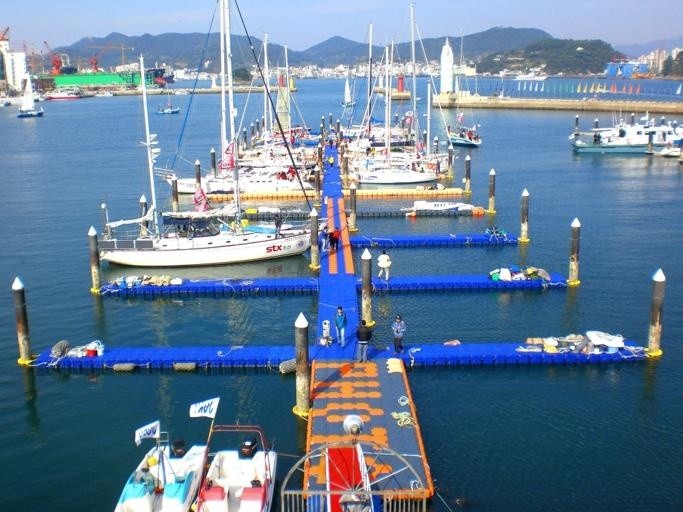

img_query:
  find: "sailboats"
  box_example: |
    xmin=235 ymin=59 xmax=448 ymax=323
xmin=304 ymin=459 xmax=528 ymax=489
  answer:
xmin=16 ymin=72 xmax=44 ymax=117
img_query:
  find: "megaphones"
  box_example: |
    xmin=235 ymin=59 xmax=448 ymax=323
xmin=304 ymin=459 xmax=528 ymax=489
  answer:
xmin=343 ymin=415 xmax=363 ymax=435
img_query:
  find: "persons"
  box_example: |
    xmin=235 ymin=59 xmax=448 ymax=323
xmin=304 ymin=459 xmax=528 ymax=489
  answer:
xmin=390 ymin=315 xmax=407 ymax=355
xmin=355 ymin=320 xmax=373 ymax=365
xmin=333 ymin=305 xmax=348 ymax=349
xmin=274 ymin=214 xmax=285 ymax=239
xmin=378 ymin=249 xmax=393 ymax=282
xmin=320 ymin=225 xmax=342 ymax=252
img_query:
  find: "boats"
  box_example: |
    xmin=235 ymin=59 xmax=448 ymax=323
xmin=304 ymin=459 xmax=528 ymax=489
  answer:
xmin=568 ymin=108 xmax=683 ymax=153
xmin=46 ymin=86 xmax=114 ymax=99
xmin=112 ymin=393 xmax=279 ymax=512
